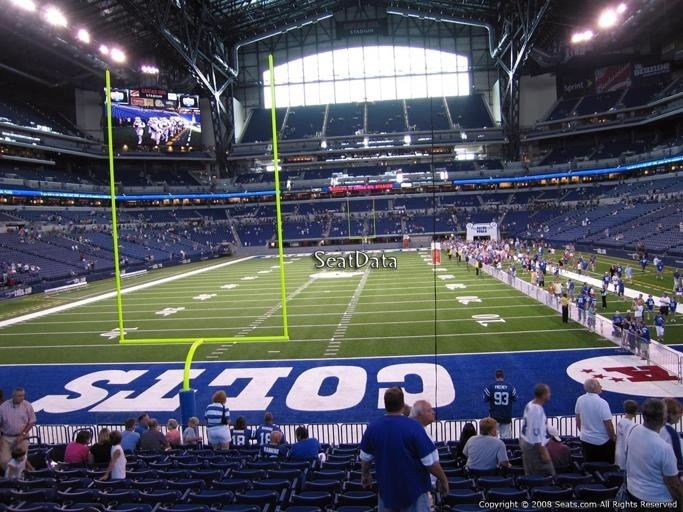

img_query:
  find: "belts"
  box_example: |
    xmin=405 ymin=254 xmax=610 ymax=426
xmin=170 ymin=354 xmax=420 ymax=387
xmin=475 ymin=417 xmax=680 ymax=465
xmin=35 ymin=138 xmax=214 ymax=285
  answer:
xmin=4 ymin=435 xmax=18 ymax=437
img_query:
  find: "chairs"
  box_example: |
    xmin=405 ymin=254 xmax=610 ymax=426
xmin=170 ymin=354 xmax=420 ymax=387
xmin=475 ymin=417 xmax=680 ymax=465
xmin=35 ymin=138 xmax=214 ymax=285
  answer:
xmin=116 ymin=152 xmax=236 ymax=267
xmin=0 ymin=84 xmax=116 ymax=295
xmin=236 ymin=93 xmax=500 ymax=241
xmin=0 ymin=422 xmax=682 ymax=512
xmin=498 ymin=60 xmax=683 ymax=268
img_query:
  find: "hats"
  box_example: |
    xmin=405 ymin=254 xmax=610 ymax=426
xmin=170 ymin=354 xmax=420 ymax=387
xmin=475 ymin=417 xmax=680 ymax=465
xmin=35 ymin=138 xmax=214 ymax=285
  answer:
xmin=547 ymin=426 xmax=562 ymax=442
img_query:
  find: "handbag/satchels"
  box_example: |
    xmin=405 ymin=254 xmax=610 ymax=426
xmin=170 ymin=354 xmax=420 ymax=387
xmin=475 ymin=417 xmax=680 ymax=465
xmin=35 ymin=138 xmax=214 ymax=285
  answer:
xmin=222 ymin=405 xmax=228 ymax=426
xmin=613 ymin=424 xmax=639 ymax=512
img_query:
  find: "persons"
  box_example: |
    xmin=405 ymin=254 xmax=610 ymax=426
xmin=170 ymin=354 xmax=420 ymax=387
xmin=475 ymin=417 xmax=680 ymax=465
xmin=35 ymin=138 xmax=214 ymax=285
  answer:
xmin=132 ymin=116 xmax=183 ymax=147
